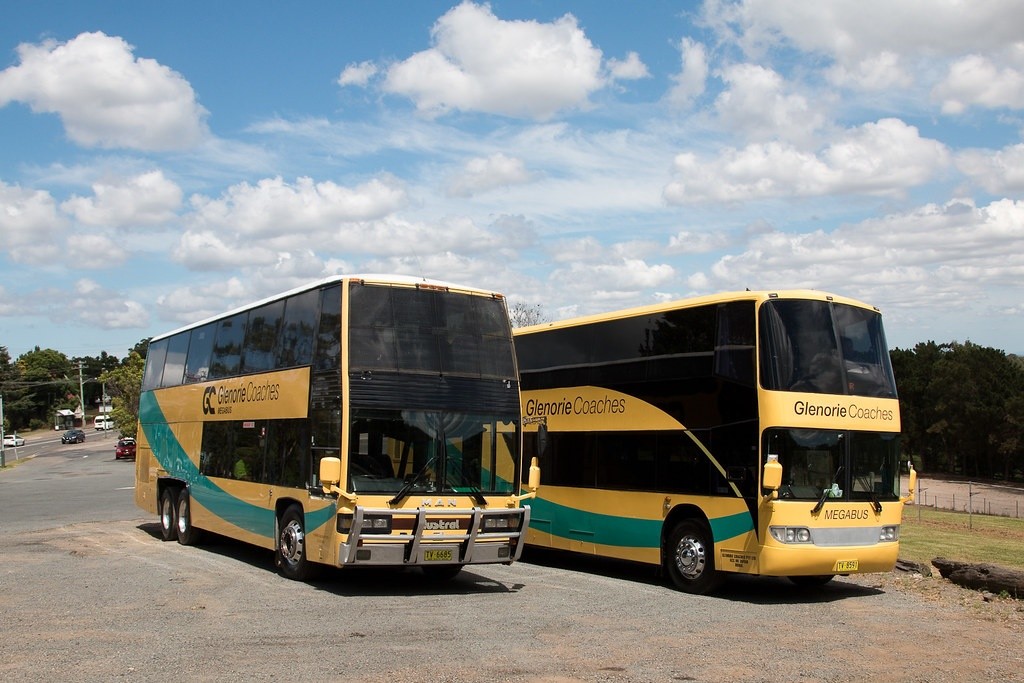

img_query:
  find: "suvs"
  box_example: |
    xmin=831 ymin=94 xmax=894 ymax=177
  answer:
xmin=61 ymin=429 xmax=86 ymax=444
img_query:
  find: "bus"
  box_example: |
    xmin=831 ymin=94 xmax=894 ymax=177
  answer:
xmin=132 ymin=272 xmax=542 ymax=584
xmin=511 ymin=289 xmax=917 ymax=595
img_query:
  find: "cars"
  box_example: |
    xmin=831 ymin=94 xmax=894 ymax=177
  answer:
xmin=3 ymin=434 xmax=25 ymax=447
xmin=114 ymin=437 xmax=137 ymax=460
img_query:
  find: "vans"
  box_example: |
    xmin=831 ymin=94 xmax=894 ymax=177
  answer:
xmin=94 ymin=415 xmax=115 ymax=431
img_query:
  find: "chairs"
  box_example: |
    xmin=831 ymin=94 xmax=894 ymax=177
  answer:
xmin=352 ymin=453 xmax=395 ymax=479
xmin=204 ymin=445 xmax=279 ymax=485
